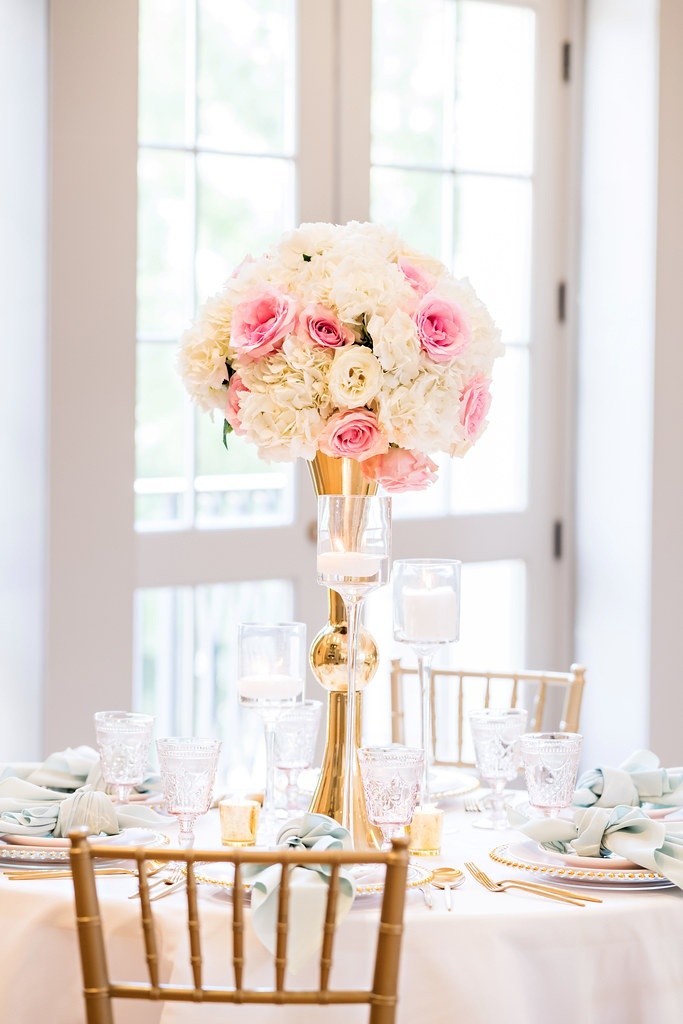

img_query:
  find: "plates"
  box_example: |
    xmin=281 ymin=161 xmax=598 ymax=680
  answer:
xmin=490 ymin=803 xmax=681 ymax=891
xmin=294 ymin=767 xmax=478 ymax=803
xmin=181 ymin=864 xmax=434 ymax=908
xmin=0 ymin=784 xmax=170 ymax=863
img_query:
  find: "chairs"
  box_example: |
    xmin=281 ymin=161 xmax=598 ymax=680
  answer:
xmin=390 ymin=659 xmax=588 ymax=774
xmin=64 ymin=826 xmax=414 ymax=1024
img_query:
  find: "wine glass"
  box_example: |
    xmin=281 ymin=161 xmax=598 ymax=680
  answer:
xmin=357 ymin=750 xmax=424 ymax=849
xmin=469 ymin=710 xmax=528 ymax=803
xmin=521 ymin=732 xmax=581 ymax=817
xmin=238 ymin=622 xmax=323 ymax=838
xmin=94 ymin=710 xmax=154 ymax=805
xmin=157 ymin=736 xmax=221 ymax=850
xmin=313 ymin=494 xmax=393 ymax=850
xmin=390 ymin=559 xmax=460 ymax=808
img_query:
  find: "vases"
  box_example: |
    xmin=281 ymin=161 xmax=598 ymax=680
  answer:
xmin=307 ymin=450 xmax=384 ymax=852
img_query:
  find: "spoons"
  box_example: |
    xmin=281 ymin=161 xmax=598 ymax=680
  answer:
xmin=431 ymin=867 xmax=465 ymax=910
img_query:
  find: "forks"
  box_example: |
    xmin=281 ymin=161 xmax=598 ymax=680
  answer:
xmin=463 ymin=793 xmax=489 ymax=812
xmin=129 ymin=866 xmax=184 ymax=899
xmin=464 ymin=862 xmax=603 ymax=907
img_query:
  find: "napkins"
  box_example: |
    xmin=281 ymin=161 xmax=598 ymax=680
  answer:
xmin=0 ymin=745 xmax=179 ymax=838
xmin=569 ymin=749 xmax=683 ymax=806
xmin=241 ymin=812 xmax=356 ymax=973
xmin=518 ymin=804 xmax=683 ymax=890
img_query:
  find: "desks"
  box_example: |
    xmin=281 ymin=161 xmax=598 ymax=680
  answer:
xmin=0 ymin=767 xmax=683 ymax=1024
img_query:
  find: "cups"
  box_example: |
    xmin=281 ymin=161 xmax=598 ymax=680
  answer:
xmin=221 ymin=801 xmax=256 ymax=845
xmin=410 ymin=808 xmax=443 ymax=854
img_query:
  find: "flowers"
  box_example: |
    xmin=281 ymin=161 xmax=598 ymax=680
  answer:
xmin=172 ymin=215 xmax=502 ymax=491
